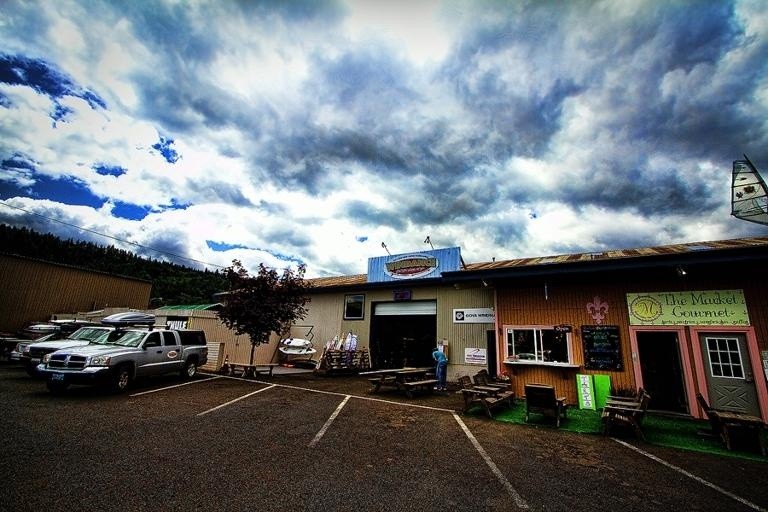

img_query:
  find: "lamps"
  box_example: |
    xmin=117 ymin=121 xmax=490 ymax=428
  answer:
xmin=423 ymin=234 xmax=436 ymax=251
xmin=380 ymin=240 xmax=392 ymax=256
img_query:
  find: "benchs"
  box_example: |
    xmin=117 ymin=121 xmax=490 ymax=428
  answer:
xmin=367 ymin=376 xmax=395 ymax=393
xmin=252 ymin=364 xmax=278 ymax=378
xmin=402 ymin=377 xmax=442 ymax=394
xmin=223 ymin=361 xmax=253 ymax=378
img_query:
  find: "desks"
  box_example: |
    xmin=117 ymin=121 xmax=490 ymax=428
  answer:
xmin=358 ymin=367 xmax=439 ymax=397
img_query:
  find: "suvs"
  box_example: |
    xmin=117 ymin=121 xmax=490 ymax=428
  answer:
xmin=0 ymin=311 xmax=208 ymax=393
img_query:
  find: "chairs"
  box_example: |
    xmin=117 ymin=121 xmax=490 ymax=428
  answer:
xmin=521 ymin=384 xmax=568 ymax=428
xmin=601 ymin=385 xmax=766 ymax=458
xmin=457 ymin=369 xmax=519 ymax=421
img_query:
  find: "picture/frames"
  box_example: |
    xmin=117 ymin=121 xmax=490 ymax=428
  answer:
xmin=343 ymin=293 xmax=366 ymax=320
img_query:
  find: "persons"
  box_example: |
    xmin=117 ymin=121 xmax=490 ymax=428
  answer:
xmin=431 ymin=344 xmax=450 ymax=392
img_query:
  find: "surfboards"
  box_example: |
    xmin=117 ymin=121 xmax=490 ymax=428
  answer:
xmin=278 ymin=329 xmax=358 ymax=372
